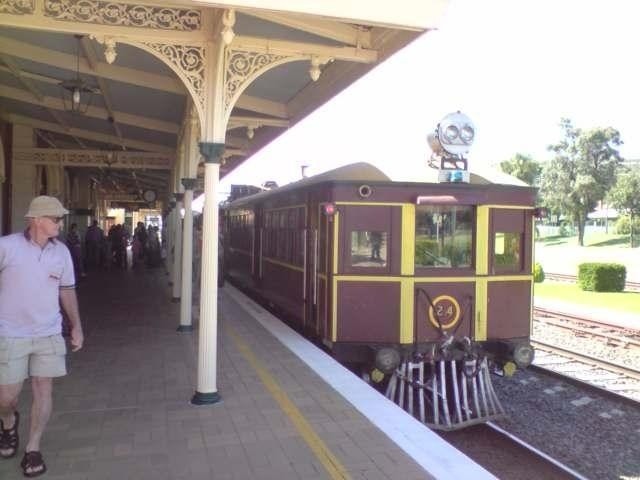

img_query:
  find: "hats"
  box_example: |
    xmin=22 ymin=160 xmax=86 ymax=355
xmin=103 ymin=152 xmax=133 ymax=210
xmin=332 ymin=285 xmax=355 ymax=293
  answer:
xmin=23 ymin=195 xmax=71 ymax=218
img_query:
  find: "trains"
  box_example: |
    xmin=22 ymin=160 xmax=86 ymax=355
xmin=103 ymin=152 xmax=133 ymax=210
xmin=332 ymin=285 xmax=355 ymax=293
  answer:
xmin=181 ymin=110 xmax=548 ymax=433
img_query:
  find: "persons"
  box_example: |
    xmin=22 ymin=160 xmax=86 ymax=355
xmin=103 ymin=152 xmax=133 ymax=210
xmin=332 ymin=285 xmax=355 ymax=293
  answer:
xmin=369 ymin=232 xmax=384 ymax=261
xmin=66 ymin=223 xmax=82 ymax=271
xmin=85 ymin=220 xmax=160 ymax=270
xmin=0 ymin=194 xmax=85 ymax=477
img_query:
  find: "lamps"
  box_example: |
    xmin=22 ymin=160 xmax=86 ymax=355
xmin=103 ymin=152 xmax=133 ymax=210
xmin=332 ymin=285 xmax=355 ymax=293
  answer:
xmin=100 ymin=114 xmax=118 ymax=173
xmin=59 ymin=35 xmax=102 ymax=124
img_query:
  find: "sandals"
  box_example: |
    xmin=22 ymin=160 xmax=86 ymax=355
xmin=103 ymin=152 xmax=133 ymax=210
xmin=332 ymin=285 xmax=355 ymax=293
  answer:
xmin=0 ymin=411 xmax=20 ymax=459
xmin=21 ymin=450 xmax=47 ymax=478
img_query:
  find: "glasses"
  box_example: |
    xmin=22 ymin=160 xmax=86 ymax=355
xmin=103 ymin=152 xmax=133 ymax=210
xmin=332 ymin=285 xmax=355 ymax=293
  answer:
xmin=40 ymin=216 xmax=63 ymax=224
